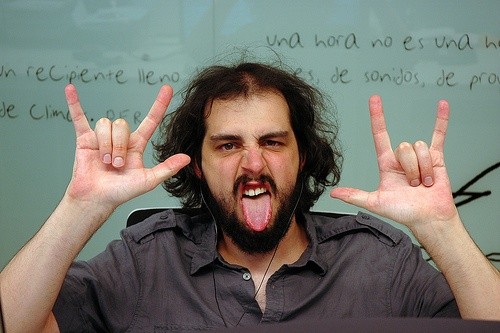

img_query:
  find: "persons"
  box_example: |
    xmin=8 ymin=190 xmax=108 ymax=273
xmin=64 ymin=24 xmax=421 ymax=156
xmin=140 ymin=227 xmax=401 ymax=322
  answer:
xmin=0 ymin=63 xmax=500 ymax=333
